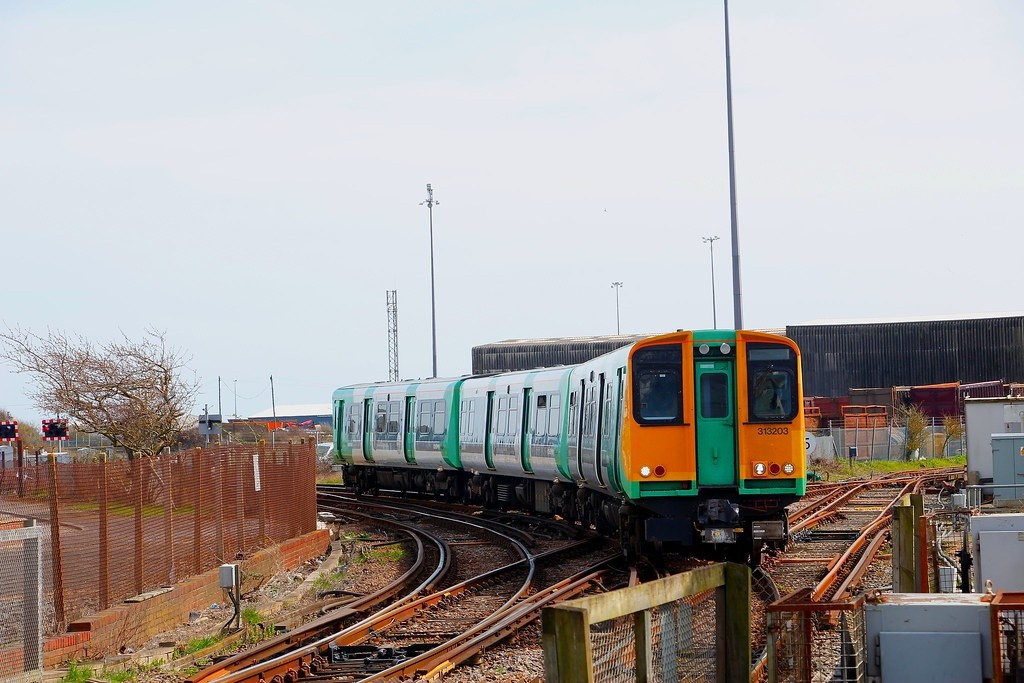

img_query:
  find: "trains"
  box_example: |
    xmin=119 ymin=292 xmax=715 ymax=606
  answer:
xmin=331 ymin=327 xmax=807 ymax=566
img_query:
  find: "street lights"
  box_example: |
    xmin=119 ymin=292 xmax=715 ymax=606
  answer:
xmin=419 ymin=183 xmax=440 ymax=377
xmin=702 ymin=235 xmax=721 ymax=330
xmin=610 ymin=282 xmax=624 ymax=335
